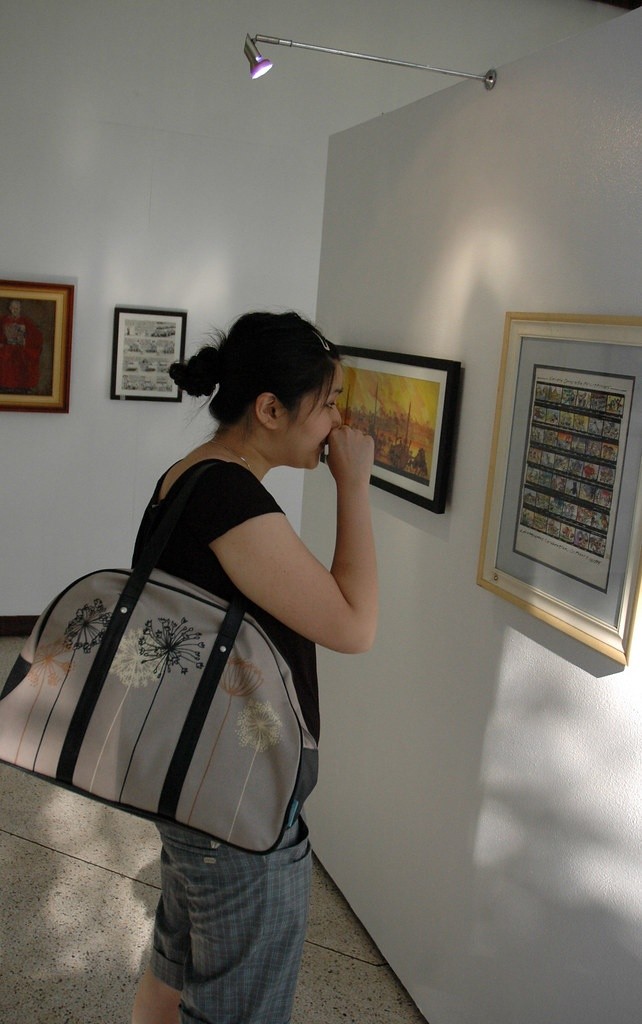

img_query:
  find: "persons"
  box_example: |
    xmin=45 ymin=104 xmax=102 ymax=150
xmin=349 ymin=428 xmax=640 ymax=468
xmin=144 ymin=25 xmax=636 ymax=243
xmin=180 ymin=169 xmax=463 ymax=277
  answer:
xmin=127 ymin=311 xmax=378 ymax=1024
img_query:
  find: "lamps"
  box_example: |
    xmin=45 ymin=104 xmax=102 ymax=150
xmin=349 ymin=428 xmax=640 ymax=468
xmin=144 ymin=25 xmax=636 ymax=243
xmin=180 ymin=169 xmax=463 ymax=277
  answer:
xmin=245 ymin=30 xmax=496 ymax=92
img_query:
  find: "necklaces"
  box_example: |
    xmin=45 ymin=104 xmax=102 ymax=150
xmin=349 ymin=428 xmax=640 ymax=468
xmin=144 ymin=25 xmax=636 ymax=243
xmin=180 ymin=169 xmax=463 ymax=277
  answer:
xmin=210 ymin=439 xmax=252 ymax=471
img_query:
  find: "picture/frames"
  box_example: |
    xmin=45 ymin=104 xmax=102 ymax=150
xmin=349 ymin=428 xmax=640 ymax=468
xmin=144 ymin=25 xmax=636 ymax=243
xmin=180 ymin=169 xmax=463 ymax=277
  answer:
xmin=0 ymin=277 xmax=75 ymax=414
xmin=475 ymin=312 xmax=642 ymax=668
xmin=318 ymin=344 xmax=465 ymax=515
xmin=109 ymin=305 xmax=188 ymax=404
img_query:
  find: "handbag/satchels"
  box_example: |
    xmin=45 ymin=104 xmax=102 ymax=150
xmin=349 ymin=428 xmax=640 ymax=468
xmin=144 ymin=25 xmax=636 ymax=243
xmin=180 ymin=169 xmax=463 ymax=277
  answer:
xmin=0 ymin=463 xmax=319 ymax=855
